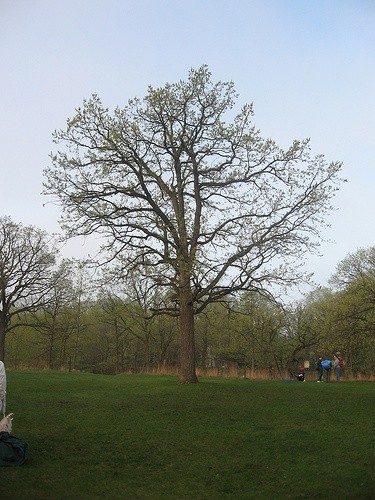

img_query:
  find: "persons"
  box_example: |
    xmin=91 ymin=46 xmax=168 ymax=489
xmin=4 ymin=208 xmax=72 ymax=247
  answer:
xmin=294 ymin=350 xmax=347 ymax=384
xmin=0 ymin=358 xmax=8 ymax=434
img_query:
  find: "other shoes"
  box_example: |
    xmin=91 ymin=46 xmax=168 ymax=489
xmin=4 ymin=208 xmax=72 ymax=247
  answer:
xmin=318 ymin=380 xmax=323 ymax=383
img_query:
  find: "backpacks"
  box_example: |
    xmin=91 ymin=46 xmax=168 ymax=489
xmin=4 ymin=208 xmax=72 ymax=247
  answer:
xmin=338 ymin=358 xmax=343 ymax=366
